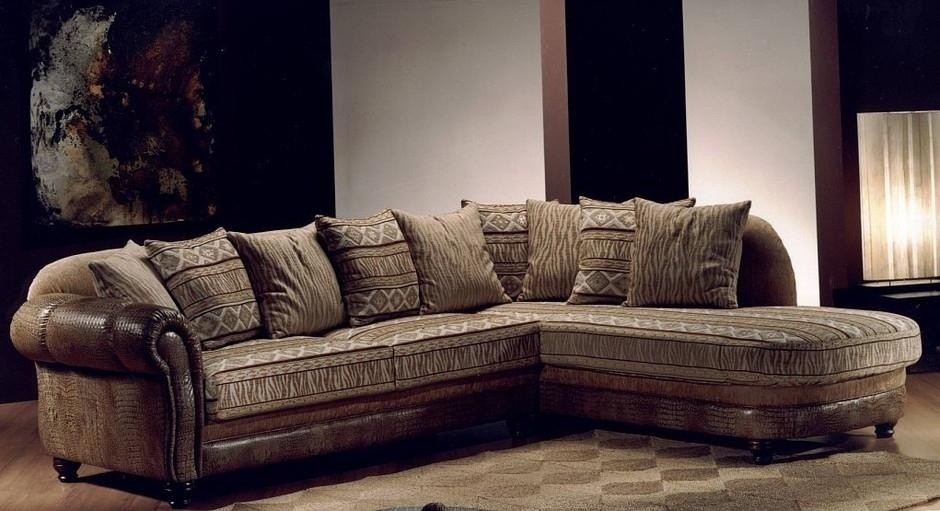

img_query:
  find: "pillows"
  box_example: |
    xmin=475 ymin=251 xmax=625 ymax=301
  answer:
xmin=86 ymin=239 xmax=182 ymax=323
xmin=226 ymin=220 xmax=345 ymax=340
xmin=144 ymin=227 xmax=263 ymax=350
xmin=567 ymin=195 xmax=695 ymax=304
xmin=623 ymin=197 xmax=751 ymax=310
xmin=389 ymin=203 xmax=512 ymax=315
xmin=314 ymin=210 xmax=422 ymax=327
xmin=519 ymin=200 xmax=635 ymax=302
xmin=459 ymin=198 xmax=558 ymax=299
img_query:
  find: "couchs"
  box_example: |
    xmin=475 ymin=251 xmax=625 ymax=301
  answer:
xmin=481 ymin=214 xmax=924 ymax=469
xmin=9 ymin=190 xmax=541 ymax=511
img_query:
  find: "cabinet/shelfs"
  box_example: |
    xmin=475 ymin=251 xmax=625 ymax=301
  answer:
xmin=877 ymin=290 xmax=939 ymax=373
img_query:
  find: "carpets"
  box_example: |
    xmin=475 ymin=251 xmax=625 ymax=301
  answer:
xmin=201 ymin=427 xmax=940 ymax=511
